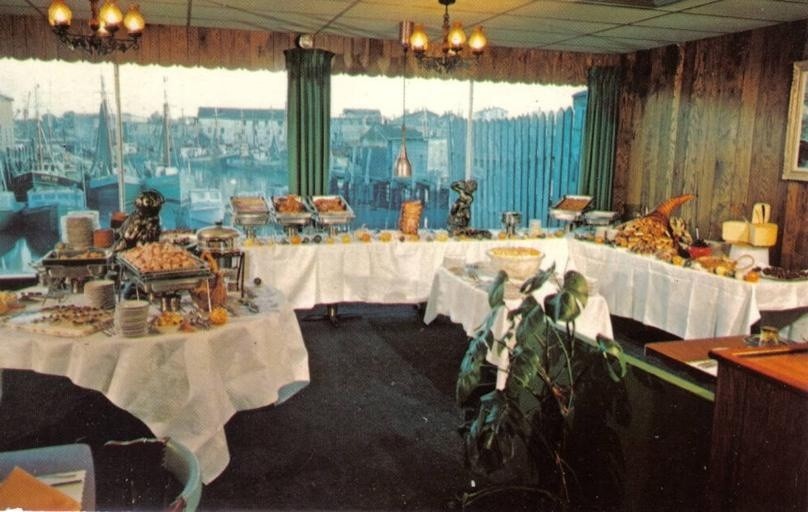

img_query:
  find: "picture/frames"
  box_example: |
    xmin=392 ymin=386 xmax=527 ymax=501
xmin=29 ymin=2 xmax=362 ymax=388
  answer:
xmin=780 ymin=57 xmax=808 ymax=186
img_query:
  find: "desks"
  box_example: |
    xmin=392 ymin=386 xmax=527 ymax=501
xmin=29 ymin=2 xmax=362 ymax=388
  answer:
xmin=436 ymin=259 xmax=604 ymax=390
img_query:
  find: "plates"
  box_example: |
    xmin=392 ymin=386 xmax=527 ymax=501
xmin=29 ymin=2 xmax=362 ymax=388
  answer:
xmin=60 ymin=209 xmax=100 ymax=250
xmin=83 ymin=280 xmax=150 ymax=337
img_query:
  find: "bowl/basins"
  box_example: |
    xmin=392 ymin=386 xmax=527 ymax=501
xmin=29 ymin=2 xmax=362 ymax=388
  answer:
xmin=486 ymin=245 xmax=546 ymax=279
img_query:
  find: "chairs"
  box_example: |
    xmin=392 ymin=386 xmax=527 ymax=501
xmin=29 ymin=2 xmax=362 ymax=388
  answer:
xmin=104 ymin=437 xmax=203 ymax=512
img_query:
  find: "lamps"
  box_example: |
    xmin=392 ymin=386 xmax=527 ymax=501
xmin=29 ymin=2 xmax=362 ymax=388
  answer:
xmin=389 ymin=20 xmax=415 ymax=181
xmin=398 ymin=0 xmax=489 ymax=69
xmin=47 ymin=0 xmax=145 ymax=60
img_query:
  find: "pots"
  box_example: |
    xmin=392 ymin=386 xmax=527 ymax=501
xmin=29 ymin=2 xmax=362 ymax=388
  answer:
xmin=195 ymin=219 xmax=244 ymax=271
